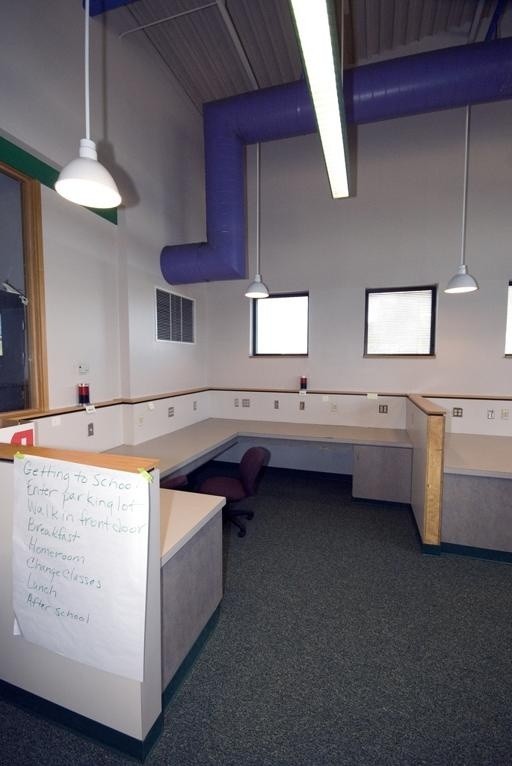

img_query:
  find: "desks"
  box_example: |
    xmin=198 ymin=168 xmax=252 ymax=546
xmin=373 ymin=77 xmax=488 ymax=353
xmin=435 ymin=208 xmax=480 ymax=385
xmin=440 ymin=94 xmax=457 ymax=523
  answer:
xmin=80 ymin=419 xmax=413 ymax=696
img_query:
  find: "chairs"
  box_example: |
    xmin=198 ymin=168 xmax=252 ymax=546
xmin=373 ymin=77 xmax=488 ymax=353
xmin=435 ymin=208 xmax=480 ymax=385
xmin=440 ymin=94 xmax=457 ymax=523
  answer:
xmin=185 ymin=446 xmax=269 ymax=540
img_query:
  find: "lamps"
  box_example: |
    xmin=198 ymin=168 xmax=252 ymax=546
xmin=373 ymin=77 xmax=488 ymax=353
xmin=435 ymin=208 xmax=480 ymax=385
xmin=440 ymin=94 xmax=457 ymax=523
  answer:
xmin=443 ymin=103 xmax=482 ymax=301
xmin=52 ymin=2 xmax=125 ymax=215
xmin=242 ymin=142 xmax=270 ymax=302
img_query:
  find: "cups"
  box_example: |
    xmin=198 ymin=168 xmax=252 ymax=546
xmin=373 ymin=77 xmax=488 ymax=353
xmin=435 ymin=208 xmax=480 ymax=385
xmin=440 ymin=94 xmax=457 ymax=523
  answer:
xmin=300 ymin=376 xmax=308 ymax=391
xmin=79 ymin=383 xmax=89 ymax=405
